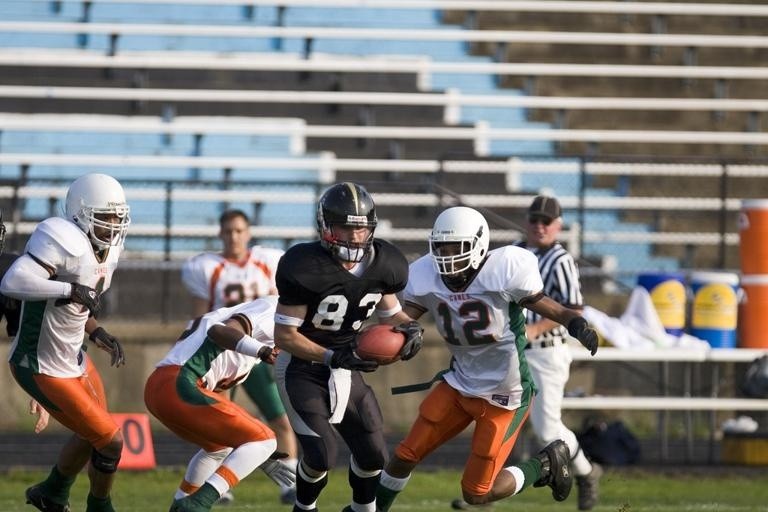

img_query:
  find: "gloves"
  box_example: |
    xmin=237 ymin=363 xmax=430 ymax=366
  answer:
xmin=259 ymin=345 xmax=282 ymax=365
xmin=572 ymin=322 xmax=598 ymax=356
xmin=70 ymin=282 xmax=102 ymax=320
xmin=260 ymin=451 xmax=296 ymax=486
xmin=88 ymin=327 xmax=126 ymax=369
xmin=330 ymin=337 xmax=377 ymax=373
xmin=392 ymin=321 xmax=424 ymax=361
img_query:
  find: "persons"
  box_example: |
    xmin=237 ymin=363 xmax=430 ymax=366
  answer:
xmin=451 ymin=195 xmax=604 ymax=511
xmin=375 ymin=207 xmax=600 ymax=511
xmin=268 ymin=177 xmax=424 ymax=512
xmin=0 ymin=172 xmax=130 ymax=512
xmin=0 ymin=223 xmax=50 ymax=436
xmin=145 ymin=290 xmax=280 ymax=512
xmin=179 ymin=207 xmax=299 ymax=503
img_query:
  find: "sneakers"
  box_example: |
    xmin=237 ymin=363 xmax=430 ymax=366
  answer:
xmin=534 ymin=438 xmax=572 ymax=502
xmin=576 ymin=462 xmax=604 ymax=510
xmin=170 ymin=497 xmax=205 ymax=512
xmin=24 ymin=483 xmax=72 ymax=512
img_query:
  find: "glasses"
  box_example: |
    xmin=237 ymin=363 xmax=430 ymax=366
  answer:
xmin=529 ymin=215 xmax=558 ymax=226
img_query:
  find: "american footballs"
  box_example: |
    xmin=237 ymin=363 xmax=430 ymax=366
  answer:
xmin=355 ymin=324 xmax=406 ymax=365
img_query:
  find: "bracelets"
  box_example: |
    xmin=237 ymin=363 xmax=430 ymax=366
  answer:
xmin=235 ymin=334 xmax=272 ymax=362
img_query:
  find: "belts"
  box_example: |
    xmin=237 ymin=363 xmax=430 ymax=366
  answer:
xmin=526 ymin=338 xmax=565 ymax=349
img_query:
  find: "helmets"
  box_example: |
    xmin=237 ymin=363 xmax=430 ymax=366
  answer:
xmin=429 ymin=206 xmax=490 ymax=274
xmin=65 ymin=173 xmax=131 ymax=250
xmin=316 ymin=182 xmax=378 ymax=263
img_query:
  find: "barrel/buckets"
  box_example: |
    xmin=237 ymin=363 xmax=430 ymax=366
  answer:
xmin=739 ymin=274 xmax=768 ymax=348
xmin=740 ymin=197 xmax=767 ymax=274
xmin=685 ymin=270 xmax=743 ymax=347
xmin=638 ymin=271 xmax=686 ymax=335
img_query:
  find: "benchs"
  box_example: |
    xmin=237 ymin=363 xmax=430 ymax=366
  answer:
xmin=0 ymin=0 xmax=768 ymax=280
xmin=519 ymin=346 xmax=767 ymax=464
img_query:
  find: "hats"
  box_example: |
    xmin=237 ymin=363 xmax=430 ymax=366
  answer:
xmin=527 ymin=196 xmax=562 ymax=219
